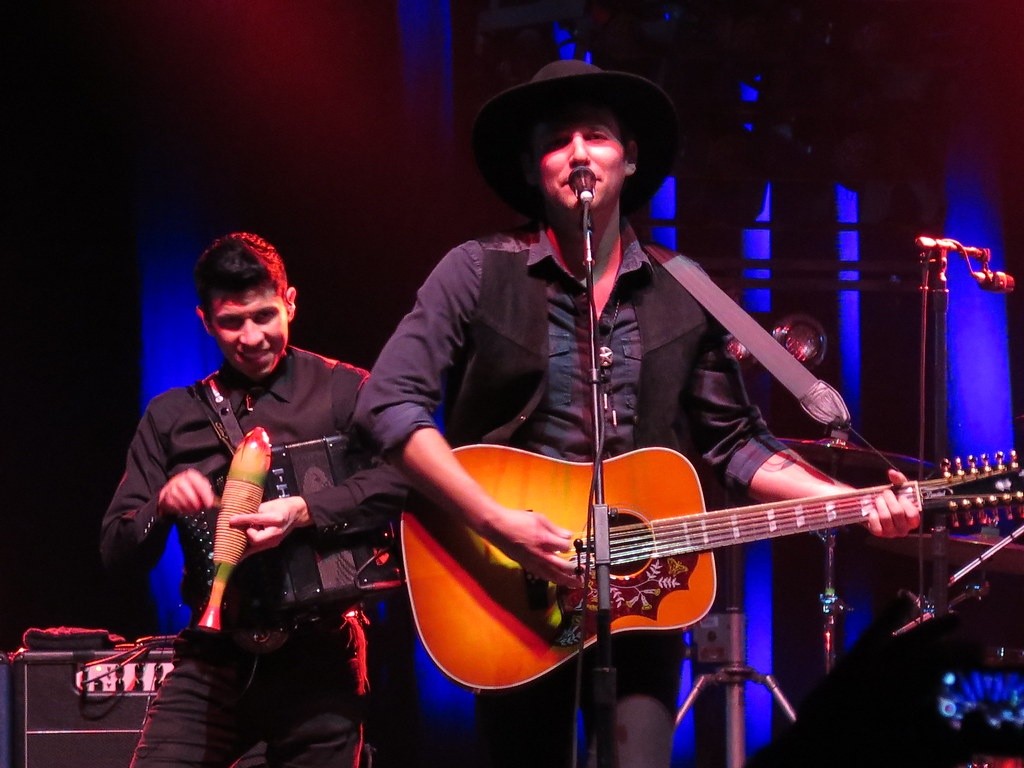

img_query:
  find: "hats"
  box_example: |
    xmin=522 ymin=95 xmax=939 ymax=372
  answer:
xmin=469 ymin=60 xmax=680 ymax=226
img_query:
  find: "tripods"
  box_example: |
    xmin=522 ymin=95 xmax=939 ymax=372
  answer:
xmin=675 ymin=477 xmax=796 ymax=768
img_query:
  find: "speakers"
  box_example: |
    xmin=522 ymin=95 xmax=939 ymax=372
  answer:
xmin=14 ymin=649 xmax=174 ymax=768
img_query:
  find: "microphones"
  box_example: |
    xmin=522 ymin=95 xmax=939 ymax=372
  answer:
xmin=972 ymin=270 xmax=1015 ymax=294
xmin=568 ymin=166 xmax=597 ymax=203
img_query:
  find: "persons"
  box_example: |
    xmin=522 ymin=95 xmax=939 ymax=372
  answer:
xmin=352 ymin=57 xmax=922 ymax=768
xmin=98 ymin=231 xmax=406 ymax=767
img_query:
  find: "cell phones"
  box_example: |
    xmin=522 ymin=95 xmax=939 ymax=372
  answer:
xmin=941 ymin=666 xmax=1024 ymax=731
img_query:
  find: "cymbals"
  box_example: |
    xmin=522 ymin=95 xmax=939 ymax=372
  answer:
xmin=874 ymin=532 xmax=1024 ymax=569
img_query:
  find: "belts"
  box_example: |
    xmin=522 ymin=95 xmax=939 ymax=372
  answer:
xmin=210 ymin=612 xmax=337 ymax=651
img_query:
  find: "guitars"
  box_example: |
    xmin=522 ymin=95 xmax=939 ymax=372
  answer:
xmin=400 ymin=444 xmax=1024 ymax=695
